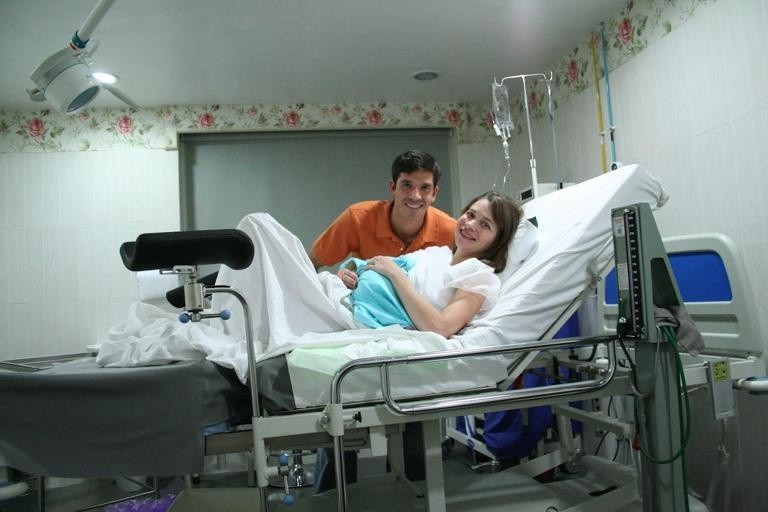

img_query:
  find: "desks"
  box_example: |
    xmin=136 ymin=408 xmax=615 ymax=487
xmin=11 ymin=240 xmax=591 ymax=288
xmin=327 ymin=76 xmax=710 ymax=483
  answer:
xmin=0 ymin=356 xmax=199 ymax=511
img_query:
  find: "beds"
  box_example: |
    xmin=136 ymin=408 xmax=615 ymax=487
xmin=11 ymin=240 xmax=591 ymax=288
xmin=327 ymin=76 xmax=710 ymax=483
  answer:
xmin=178 ymin=164 xmax=768 ymax=510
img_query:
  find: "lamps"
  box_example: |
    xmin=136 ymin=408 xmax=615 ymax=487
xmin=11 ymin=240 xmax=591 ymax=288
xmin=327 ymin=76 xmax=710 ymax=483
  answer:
xmin=29 ymin=0 xmax=118 ymax=116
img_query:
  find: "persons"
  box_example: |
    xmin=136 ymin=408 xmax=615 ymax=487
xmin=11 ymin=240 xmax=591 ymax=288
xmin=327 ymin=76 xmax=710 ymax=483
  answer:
xmin=309 ymin=149 xmax=458 ymax=495
xmin=337 ymin=190 xmax=525 ymax=341
